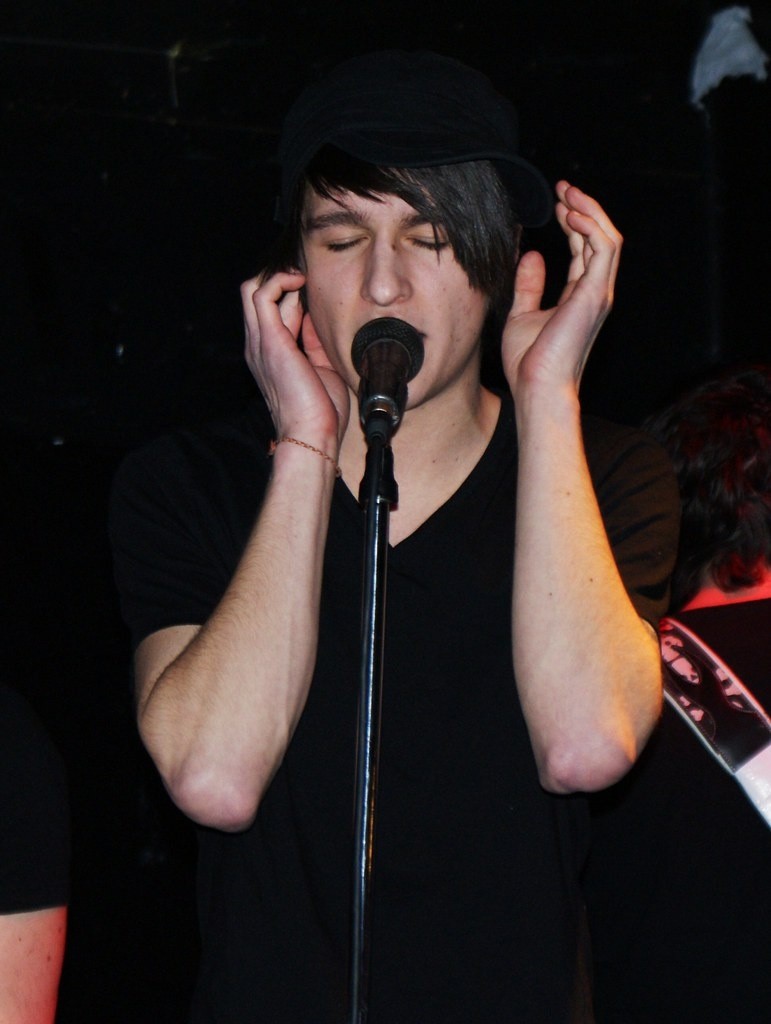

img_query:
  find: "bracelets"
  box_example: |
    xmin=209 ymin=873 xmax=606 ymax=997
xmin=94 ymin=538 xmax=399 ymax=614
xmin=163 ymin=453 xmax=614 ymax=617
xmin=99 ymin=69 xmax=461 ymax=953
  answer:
xmin=267 ymin=436 xmax=342 ymax=478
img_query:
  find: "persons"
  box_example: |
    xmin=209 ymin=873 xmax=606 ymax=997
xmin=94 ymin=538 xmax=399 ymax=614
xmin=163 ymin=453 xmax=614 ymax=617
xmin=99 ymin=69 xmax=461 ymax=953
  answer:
xmin=585 ymin=364 xmax=771 ymax=1024
xmin=130 ymin=45 xmax=666 ymax=1024
xmin=0 ymin=682 xmax=73 ymax=1024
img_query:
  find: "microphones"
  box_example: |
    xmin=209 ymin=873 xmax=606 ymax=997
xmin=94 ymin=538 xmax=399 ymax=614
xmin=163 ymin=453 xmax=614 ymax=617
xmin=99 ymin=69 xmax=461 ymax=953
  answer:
xmin=349 ymin=317 xmax=425 ymax=449
